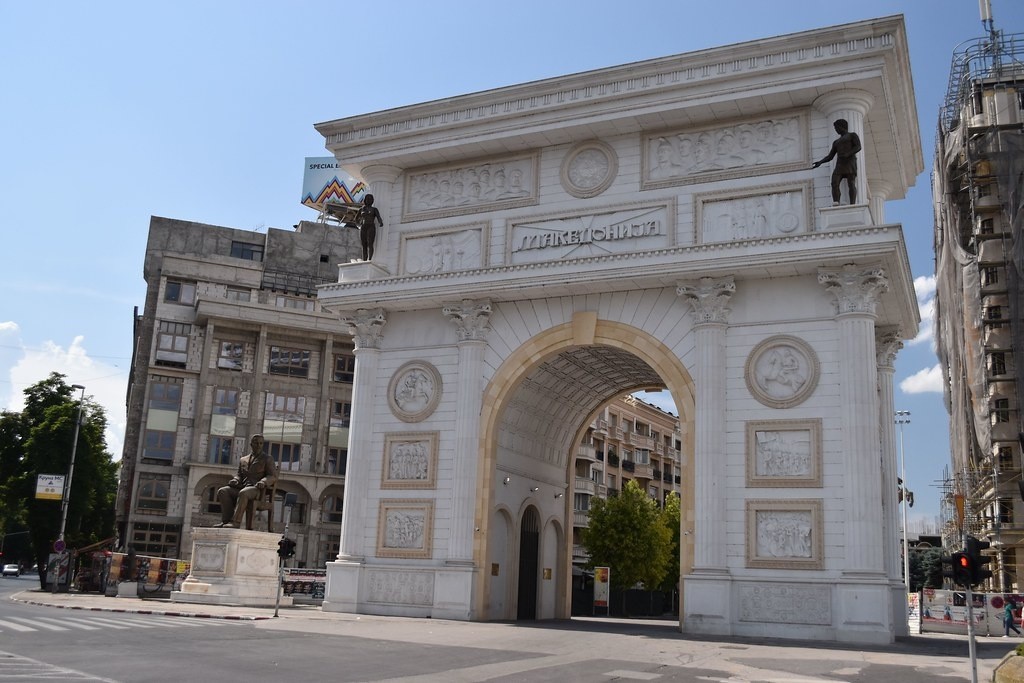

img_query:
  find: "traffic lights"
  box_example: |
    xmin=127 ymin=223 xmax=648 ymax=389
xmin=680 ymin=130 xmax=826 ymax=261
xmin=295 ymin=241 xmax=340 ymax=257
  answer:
xmin=967 ymin=534 xmax=993 ymax=587
xmin=941 ymin=551 xmax=959 ymax=585
xmin=286 ymin=539 xmax=296 ymax=559
xmin=956 ymin=555 xmax=974 ymax=586
xmin=277 ymin=539 xmax=285 ymax=556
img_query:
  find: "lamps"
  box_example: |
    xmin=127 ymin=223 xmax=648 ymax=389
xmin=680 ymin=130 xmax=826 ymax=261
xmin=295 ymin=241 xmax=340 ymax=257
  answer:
xmin=530 ymin=487 xmax=539 ymax=492
xmin=504 ymin=478 xmax=509 ymax=485
xmin=555 ymin=494 xmax=563 ymax=498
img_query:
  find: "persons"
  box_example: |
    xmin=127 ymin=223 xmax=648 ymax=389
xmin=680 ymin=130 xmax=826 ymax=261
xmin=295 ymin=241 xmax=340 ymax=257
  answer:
xmin=811 ymin=119 xmax=862 ymax=205
xmin=212 ymin=433 xmax=278 ymax=529
xmin=350 ymin=194 xmax=384 ymax=261
xmin=1003 ymin=598 xmax=1022 ymax=638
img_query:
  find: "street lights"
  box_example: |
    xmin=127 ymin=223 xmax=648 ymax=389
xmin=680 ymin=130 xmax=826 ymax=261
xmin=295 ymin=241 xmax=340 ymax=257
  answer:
xmin=894 ymin=411 xmax=913 ymax=599
xmin=51 ymin=385 xmax=86 ymax=592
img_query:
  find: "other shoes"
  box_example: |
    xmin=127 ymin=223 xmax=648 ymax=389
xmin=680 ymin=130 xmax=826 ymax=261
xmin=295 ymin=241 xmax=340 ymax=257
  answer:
xmin=1002 ymin=634 xmax=1009 ymax=637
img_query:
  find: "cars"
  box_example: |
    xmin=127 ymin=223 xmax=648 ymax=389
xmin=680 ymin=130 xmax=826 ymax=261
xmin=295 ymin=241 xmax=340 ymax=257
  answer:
xmin=19 ymin=565 xmax=24 ymax=574
xmin=2 ymin=564 xmax=21 ymax=578
xmin=32 ymin=562 xmax=47 ymax=572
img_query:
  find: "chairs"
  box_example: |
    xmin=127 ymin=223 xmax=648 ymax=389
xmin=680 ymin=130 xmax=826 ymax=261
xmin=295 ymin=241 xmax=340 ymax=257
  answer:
xmin=246 ymin=466 xmax=279 ymax=532
